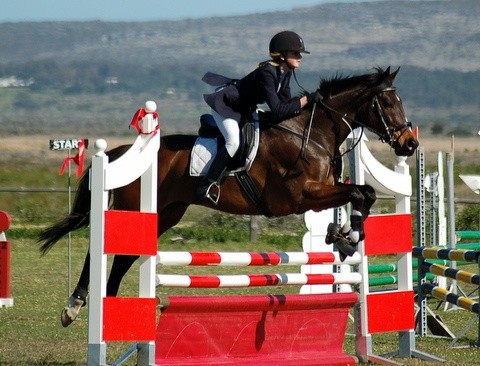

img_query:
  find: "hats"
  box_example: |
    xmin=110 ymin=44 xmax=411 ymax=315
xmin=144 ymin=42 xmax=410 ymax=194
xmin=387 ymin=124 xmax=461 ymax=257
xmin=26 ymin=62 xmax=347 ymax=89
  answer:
xmin=268 ymin=31 xmax=310 ymax=55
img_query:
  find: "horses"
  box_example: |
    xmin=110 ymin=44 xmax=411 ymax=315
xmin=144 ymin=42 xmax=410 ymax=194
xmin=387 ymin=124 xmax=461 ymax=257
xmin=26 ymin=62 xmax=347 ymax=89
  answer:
xmin=34 ymin=64 xmax=420 ymax=328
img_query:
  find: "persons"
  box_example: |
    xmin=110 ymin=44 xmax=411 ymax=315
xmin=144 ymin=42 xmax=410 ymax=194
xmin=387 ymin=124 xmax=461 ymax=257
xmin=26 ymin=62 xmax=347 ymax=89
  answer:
xmin=190 ymin=31 xmax=323 ymax=205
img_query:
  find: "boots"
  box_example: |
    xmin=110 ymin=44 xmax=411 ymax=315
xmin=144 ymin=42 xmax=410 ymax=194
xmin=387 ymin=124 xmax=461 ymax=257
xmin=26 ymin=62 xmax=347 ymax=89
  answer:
xmin=188 ymin=145 xmax=233 ymax=203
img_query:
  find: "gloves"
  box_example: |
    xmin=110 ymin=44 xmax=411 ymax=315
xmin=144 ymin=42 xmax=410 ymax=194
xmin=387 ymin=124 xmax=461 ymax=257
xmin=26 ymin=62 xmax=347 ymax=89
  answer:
xmin=306 ymin=92 xmax=323 ymax=104
